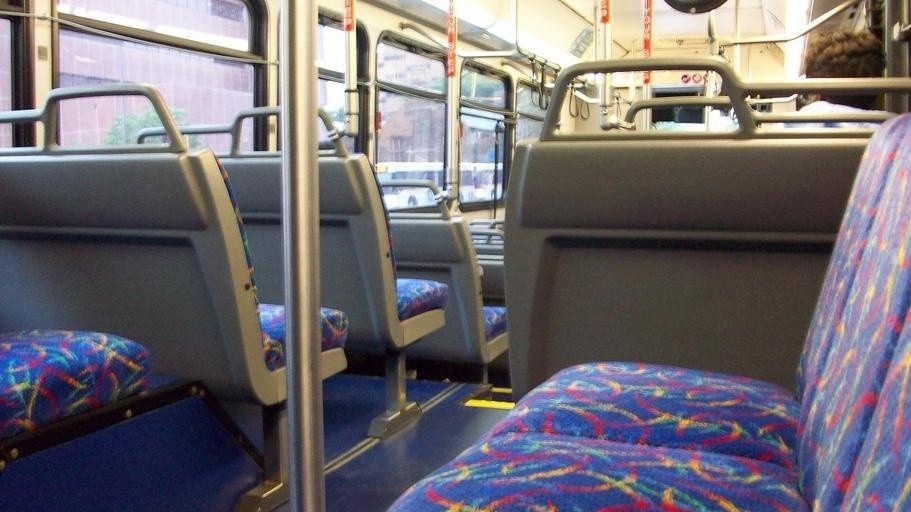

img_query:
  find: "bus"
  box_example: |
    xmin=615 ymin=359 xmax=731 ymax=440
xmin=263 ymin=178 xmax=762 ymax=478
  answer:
xmin=372 ymin=159 xmax=503 ymax=209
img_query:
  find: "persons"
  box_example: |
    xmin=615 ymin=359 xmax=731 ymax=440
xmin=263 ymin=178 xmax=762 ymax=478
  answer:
xmin=769 ymin=24 xmax=888 ymax=129
